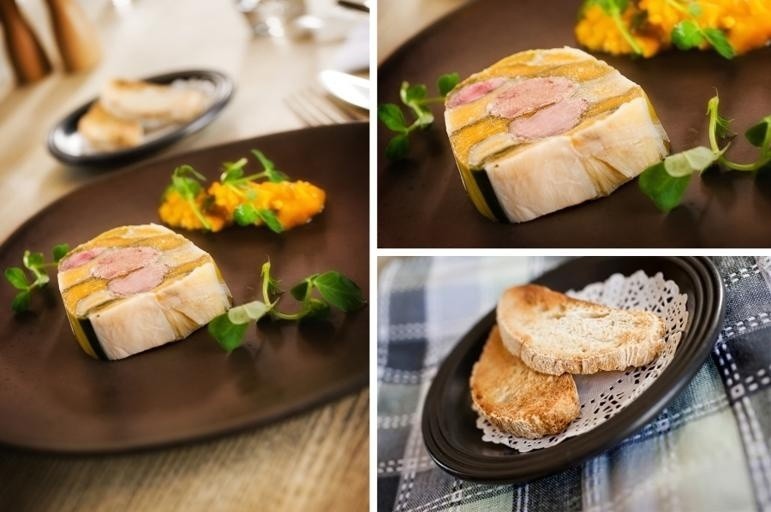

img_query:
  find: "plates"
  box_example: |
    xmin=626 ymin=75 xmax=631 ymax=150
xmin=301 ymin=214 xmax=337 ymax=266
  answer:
xmin=43 ymin=67 xmax=239 ymax=176
xmin=0 ymin=119 xmax=370 ymax=459
xmin=419 ymin=256 xmax=727 ymax=485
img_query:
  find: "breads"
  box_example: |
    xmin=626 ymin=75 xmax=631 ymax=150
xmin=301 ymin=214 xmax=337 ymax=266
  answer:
xmin=496 ymin=283 xmax=665 ymax=377
xmin=99 ymin=80 xmax=203 ymax=121
xmin=79 ymin=101 xmax=148 ymax=150
xmin=470 ymin=324 xmax=580 ymax=438
